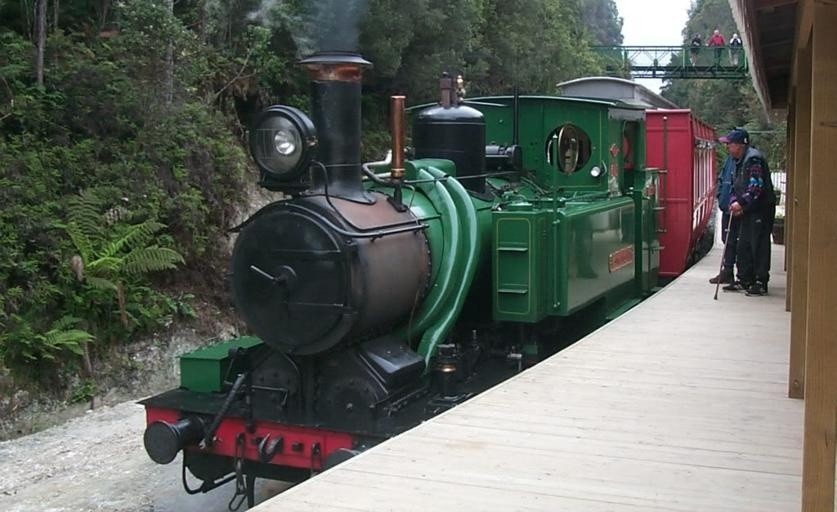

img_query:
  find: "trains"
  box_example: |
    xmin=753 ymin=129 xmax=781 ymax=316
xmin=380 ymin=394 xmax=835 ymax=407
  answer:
xmin=135 ymin=52 xmax=717 ymax=512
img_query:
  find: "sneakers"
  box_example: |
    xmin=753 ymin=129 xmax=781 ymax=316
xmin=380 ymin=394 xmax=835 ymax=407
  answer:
xmin=721 ymin=281 xmax=748 ymax=292
xmin=744 ymin=279 xmax=767 ymax=296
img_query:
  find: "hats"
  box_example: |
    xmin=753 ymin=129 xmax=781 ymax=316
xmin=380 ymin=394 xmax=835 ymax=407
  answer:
xmin=717 ymin=130 xmax=748 ymax=145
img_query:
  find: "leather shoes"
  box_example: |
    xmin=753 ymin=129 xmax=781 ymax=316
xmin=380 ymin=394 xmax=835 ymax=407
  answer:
xmin=709 ymin=271 xmax=734 ymax=284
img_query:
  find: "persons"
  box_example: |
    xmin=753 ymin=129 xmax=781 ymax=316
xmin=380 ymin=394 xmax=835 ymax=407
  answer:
xmin=690 ymin=32 xmax=702 ymax=66
xmin=708 ymin=155 xmax=742 ymax=285
xmin=718 ymin=127 xmax=778 ymax=296
xmin=728 ymin=33 xmax=741 ymax=65
xmin=705 ymin=29 xmax=726 ymax=64
